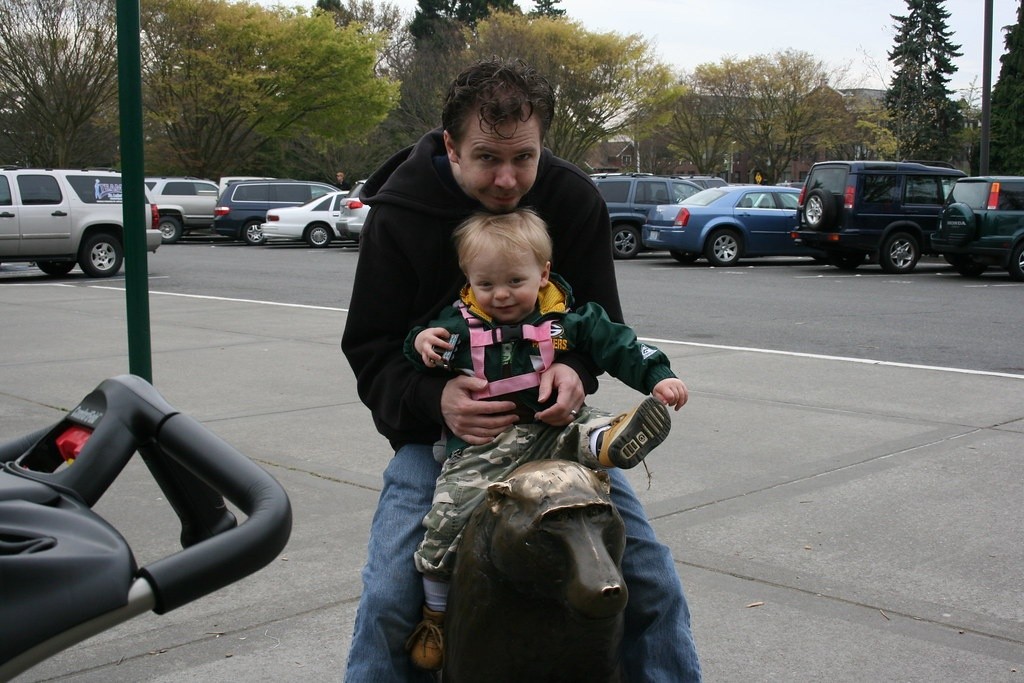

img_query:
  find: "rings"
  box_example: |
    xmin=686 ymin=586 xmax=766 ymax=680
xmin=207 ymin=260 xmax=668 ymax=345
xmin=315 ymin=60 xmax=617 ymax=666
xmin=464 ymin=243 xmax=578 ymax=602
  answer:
xmin=570 ymin=409 xmax=580 ymax=420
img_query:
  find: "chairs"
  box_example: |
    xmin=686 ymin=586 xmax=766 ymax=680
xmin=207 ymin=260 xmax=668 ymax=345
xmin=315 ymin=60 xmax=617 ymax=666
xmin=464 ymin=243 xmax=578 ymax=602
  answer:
xmin=743 ymin=198 xmax=771 ymax=208
xmin=655 ymin=191 xmax=669 ymax=203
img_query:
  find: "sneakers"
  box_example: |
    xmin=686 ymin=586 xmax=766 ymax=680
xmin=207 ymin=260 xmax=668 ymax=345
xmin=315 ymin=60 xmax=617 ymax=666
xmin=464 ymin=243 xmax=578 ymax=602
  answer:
xmin=404 ymin=605 xmax=446 ymax=672
xmin=598 ymin=395 xmax=672 ymax=469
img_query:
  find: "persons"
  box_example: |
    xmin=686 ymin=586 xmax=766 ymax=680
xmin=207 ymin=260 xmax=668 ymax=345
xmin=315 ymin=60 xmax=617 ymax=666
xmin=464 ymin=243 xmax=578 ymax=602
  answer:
xmin=402 ymin=209 xmax=688 ymax=668
xmin=341 ymin=63 xmax=702 ymax=683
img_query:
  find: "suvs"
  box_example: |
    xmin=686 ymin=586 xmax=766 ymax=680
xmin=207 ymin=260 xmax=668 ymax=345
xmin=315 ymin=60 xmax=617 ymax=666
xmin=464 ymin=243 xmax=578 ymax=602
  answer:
xmin=0 ymin=162 xmax=162 ymax=278
xmin=790 ymin=159 xmax=971 ymax=275
xmin=929 ymin=175 xmax=1024 ymax=280
xmin=218 ymin=176 xmax=279 ymax=201
xmin=334 ymin=179 xmax=371 ymax=242
xmin=208 ymin=179 xmax=343 ymax=246
xmin=587 ymin=171 xmax=731 ymax=259
xmin=144 ymin=175 xmax=220 ymax=244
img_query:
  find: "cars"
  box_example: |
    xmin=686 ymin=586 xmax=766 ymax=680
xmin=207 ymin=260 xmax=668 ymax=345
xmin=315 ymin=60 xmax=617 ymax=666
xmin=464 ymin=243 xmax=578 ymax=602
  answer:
xmin=639 ymin=184 xmax=831 ymax=267
xmin=260 ymin=190 xmax=350 ymax=249
